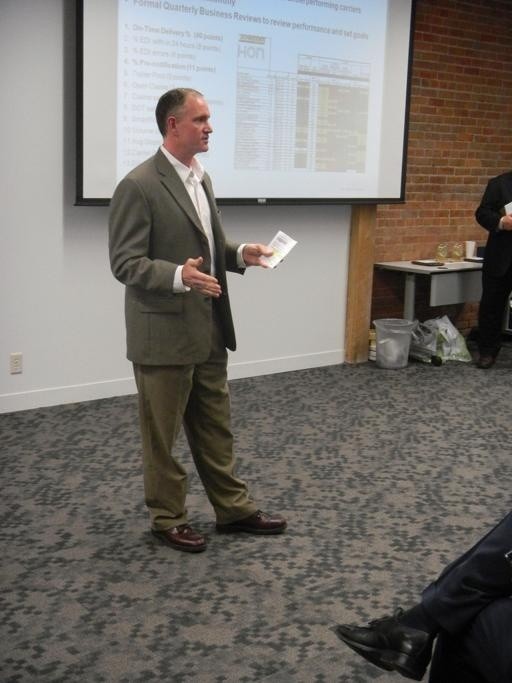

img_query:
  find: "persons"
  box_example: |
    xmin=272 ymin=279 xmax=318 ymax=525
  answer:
xmin=475 ymin=171 xmax=512 ymax=369
xmin=334 ymin=510 xmax=512 ymax=683
xmin=108 ymin=88 xmax=287 ymax=553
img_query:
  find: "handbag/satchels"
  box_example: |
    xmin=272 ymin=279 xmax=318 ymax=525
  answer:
xmin=412 ymin=314 xmax=472 ymax=363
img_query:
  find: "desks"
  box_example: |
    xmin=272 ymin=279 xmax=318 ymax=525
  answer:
xmin=374 ymin=255 xmax=484 ymax=366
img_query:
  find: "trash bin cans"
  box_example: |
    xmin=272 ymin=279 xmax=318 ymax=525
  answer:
xmin=372 ymin=318 xmax=414 ymax=369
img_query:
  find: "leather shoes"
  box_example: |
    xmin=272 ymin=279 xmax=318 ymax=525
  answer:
xmin=151 ymin=523 xmax=207 ymax=554
xmin=335 ymin=617 xmax=434 ymax=681
xmin=477 ymin=354 xmax=498 ymax=370
xmin=215 ymin=511 xmax=287 ymax=535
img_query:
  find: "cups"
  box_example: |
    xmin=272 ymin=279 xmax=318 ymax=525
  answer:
xmin=465 ymin=241 xmax=475 ymax=260
